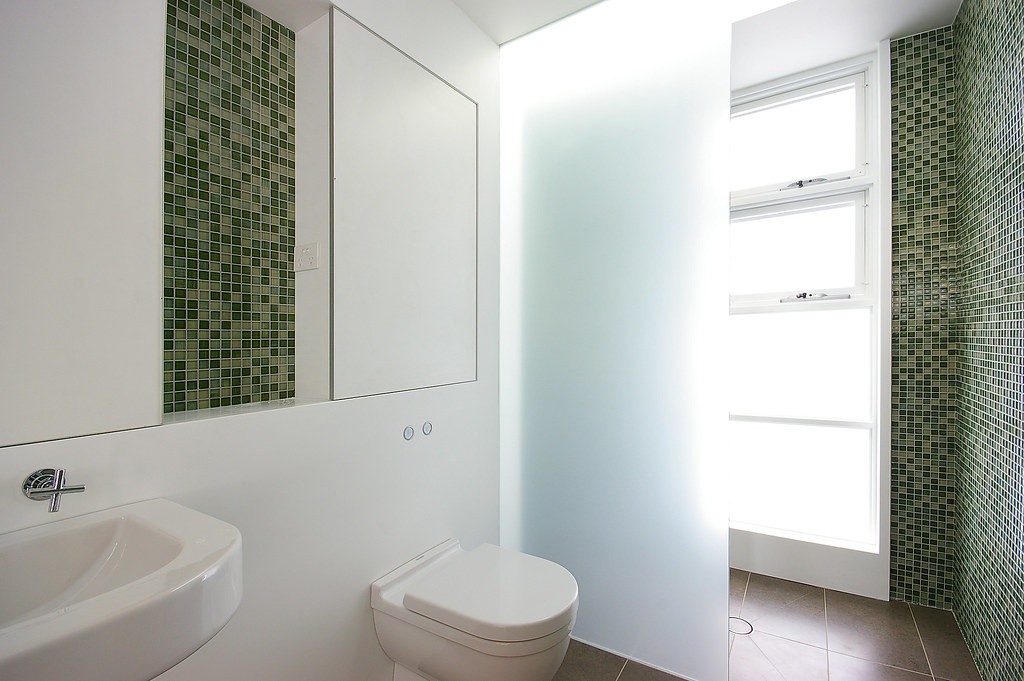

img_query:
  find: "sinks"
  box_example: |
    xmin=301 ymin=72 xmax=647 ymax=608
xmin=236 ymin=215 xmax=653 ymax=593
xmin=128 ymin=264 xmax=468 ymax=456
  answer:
xmin=0 ymin=497 xmax=244 ymax=681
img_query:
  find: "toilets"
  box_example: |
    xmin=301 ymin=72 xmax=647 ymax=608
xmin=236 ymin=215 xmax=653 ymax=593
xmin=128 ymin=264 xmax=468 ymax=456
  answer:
xmin=370 ymin=536 xmax=579 ymax=681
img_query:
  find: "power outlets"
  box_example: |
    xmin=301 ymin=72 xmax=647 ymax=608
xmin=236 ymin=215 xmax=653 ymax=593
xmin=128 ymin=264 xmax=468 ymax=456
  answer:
xmin=293 ymin=242 xmax=318 ymax=272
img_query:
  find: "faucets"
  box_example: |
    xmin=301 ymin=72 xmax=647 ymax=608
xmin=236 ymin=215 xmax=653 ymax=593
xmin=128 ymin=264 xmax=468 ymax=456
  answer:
xmin=26 ymin=469 xmax=86 ymax=512
